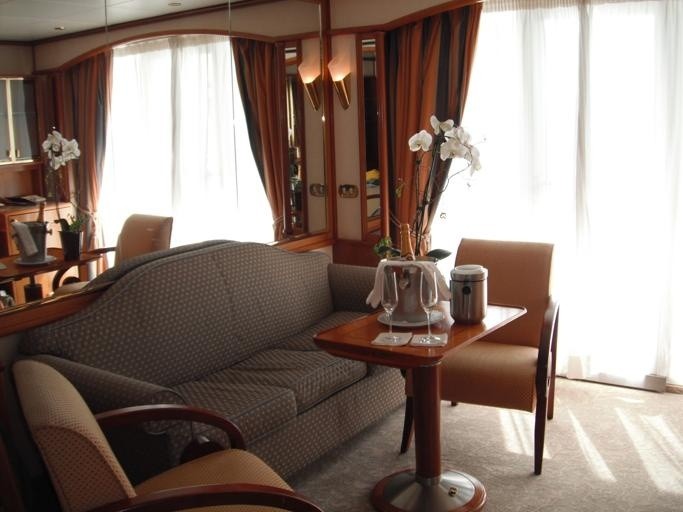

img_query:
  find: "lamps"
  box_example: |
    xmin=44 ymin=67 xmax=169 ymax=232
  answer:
xmin=327 ymin=54 xmax=351 ymax=110
xmin=298 ymin=55 xmax=322 ymax=111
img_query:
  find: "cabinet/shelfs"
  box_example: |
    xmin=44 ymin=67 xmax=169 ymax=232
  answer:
xmin=0 ymin=73 xmax=81 ymax=305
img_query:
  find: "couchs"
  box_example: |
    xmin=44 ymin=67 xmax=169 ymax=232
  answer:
xmin=78 ymin=239 xmax=236 ymax=289
xmin=17 ymin=241 xmax=407 ymax=510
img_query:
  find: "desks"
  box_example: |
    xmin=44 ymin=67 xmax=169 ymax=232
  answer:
xmin=0 ymin=248 xmax=101 ymax=303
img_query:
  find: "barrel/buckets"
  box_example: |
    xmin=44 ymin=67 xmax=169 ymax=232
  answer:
xmin=384 ymin=264 xmax=437 ymax=321
xmin=12 ymin=221 xmax=52 ymax=262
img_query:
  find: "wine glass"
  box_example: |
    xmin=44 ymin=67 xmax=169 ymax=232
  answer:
xmin=418 ymin=270 xmax=440 ymax=343
xmin=377 ymin=270 xmax=400 ymax=345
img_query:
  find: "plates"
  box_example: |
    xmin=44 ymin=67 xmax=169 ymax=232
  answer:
xmin=2 ymin=196 xmax=38 ymax=206
xmin=375 ymin=311 xmax=445 ymax=328
xmin=13 ymin=253 xmax=56 ymax=266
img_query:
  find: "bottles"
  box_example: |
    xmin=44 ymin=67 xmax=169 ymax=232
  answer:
xmin=398 ymin=222 xmax=416 ymax=261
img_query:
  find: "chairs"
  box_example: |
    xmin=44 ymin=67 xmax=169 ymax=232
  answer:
xmin=52 ymin=213 xmax=173 ymax=296
xmin=399 ymin=238 xmax=559 ymax=476
xmin=0 ymin=360 xmax=324 ymax=512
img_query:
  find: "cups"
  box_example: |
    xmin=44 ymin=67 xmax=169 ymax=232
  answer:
xmin=448 ymin=264 xmax=488 ymax=325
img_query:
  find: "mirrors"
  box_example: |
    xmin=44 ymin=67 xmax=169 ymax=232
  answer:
xmin=0 ymin=0 xmax=337 ymax=337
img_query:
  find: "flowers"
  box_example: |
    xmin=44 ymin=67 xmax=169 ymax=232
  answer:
xmin=41 ymin=131 xmax=92 ymax=231
xmin=408 ymin=115 xmax=482 ymax=256
